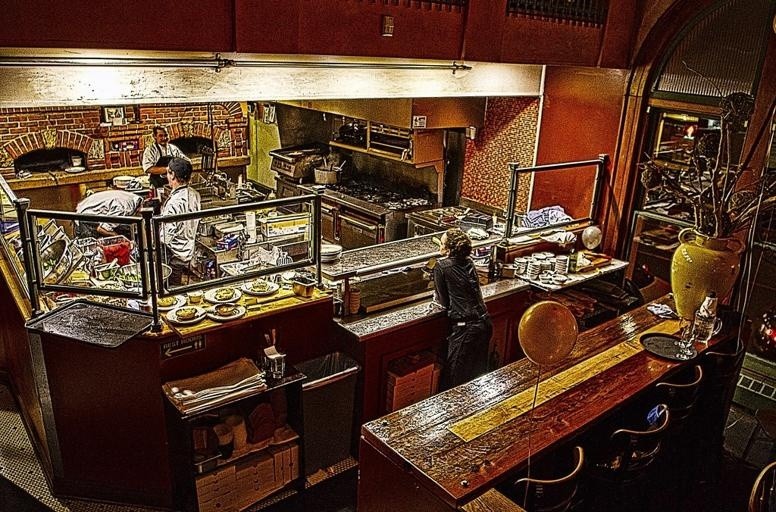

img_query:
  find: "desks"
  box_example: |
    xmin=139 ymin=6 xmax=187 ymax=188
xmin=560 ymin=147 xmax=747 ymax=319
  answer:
xmin=348 ymin=291 xmax=749 ymax=510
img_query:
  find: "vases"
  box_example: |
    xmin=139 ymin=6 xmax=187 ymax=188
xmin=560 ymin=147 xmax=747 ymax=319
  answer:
xmin=669 ymin=227 xmax=747 ymax=321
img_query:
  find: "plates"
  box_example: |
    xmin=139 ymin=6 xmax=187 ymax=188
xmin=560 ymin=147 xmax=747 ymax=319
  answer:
xmin=65 ymin=166 xmax=85 ymax=174
xmin=312 ymin=244 xmax=344 ymax=264
xmin=159 ymin=273 xmax=312 ymax=326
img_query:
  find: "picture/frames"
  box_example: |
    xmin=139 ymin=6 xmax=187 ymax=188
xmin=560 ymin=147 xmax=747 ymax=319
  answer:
xmin=100 ymin=106 xmax=125 ymax=124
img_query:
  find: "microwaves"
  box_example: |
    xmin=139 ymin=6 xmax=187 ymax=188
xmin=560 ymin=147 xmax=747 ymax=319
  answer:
xmin=369 ymin=124 xmax=412 ymax=162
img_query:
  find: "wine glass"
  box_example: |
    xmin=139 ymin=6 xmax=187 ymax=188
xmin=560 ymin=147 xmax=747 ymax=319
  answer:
xmin=681 ymin=318 xmax=696 ymax=360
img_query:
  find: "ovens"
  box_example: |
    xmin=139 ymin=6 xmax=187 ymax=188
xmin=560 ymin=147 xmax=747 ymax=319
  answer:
xmin=320 ymin=188 xmax=384 ymax=250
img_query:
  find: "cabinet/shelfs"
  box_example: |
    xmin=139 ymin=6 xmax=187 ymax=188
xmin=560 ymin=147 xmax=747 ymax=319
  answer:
xmin=329 ymin=116 xmax=445 ymax=166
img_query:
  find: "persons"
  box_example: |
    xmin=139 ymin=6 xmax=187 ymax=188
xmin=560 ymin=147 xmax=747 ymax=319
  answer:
xmin=433 ymin=229 xmax=493 ymax=386
xmin=158 ymin=156 xmax=201 ymax=285
xmin=140 ymin=125 xmax=192 ymax=187
xmin=72 ymin=192 xmax=158 ymax=241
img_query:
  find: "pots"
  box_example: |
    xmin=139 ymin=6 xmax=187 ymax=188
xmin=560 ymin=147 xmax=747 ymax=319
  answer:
xmin=313 ymin=166 xmax=342 ymax=186
xmin=5 ymin=216 xmax=82 ymax=291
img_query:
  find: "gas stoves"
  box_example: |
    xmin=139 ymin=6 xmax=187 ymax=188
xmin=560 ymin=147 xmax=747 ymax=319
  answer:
xmin=329 ymin=180 xmax=436 ymax=213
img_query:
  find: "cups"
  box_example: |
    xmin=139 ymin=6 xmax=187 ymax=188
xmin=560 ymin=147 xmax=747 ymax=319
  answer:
xmin=693 ymin=311 xmax=723 ymax=342
